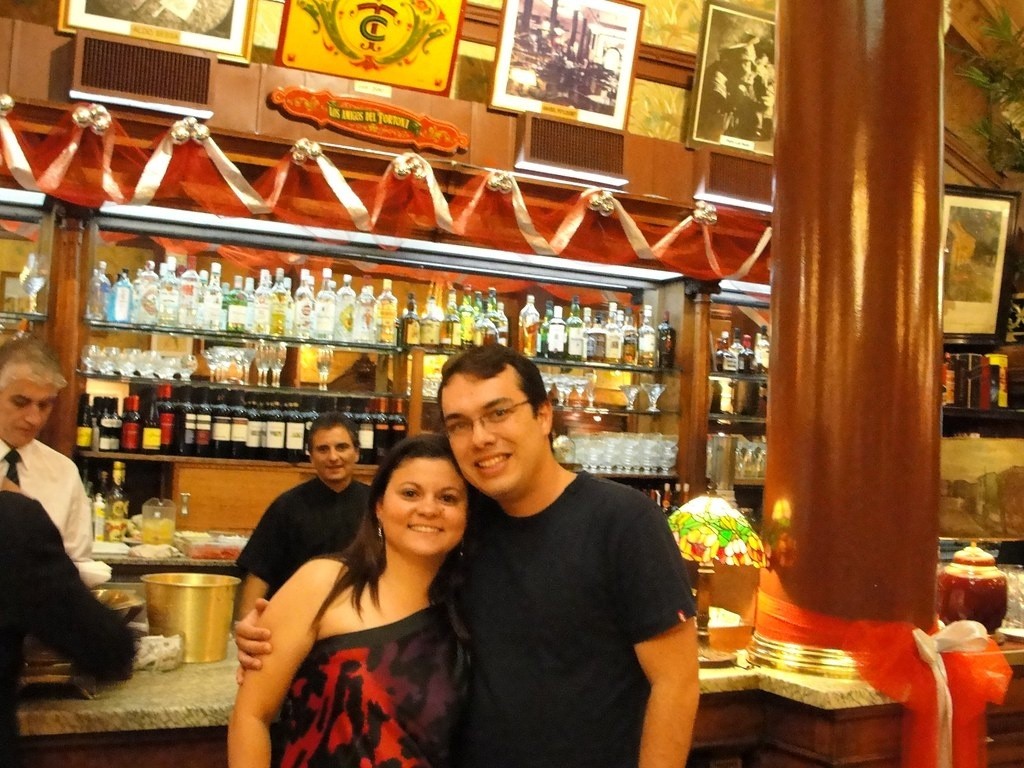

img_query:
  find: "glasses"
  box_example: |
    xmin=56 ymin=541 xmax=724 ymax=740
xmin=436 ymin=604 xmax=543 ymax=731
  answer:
xmin=444 ymin=399 xmax=530 ymax=440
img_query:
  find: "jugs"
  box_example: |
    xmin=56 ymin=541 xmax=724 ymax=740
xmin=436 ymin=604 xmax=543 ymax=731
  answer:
xmin=141 ymin=497 xmax=176 ymax=548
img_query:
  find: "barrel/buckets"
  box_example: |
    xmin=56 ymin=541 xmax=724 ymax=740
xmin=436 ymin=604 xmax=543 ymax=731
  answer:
xmin=141 ymin=573 xmax=241 ymax=664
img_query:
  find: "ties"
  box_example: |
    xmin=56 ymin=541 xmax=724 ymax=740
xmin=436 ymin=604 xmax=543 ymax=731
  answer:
xmin=4 ymin=450 xmax=21 ymax=489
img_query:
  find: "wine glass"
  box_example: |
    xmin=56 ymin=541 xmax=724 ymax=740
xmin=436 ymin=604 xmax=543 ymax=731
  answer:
xmin=81 ymin=343 xmax=197 ymax=381
xmin=556 ymin=430 xmax=679 ymax=476
xmin=735 ymin=441 xmax=766 ymax=478
xmin=203 ymin=341 xmax=288 ymax=389
xmin=620 ymin=384 xmax=641 ymax=411
xmin=540 ymin=372 xmax=598 ymax=410
xmin=17 ymin=251 xmax=47 ymax=315
xmin=641 ymin=381 xmax=667 ymax=412
xmin=314 ymin=348 xmax=334 ymax=391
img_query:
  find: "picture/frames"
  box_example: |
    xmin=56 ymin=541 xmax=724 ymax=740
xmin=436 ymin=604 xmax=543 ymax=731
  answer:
xmin=686 ymin=0 xmax=777 ymax=164
xmin=938 ymin=184 xmax=1022 ymax=346
xmin=488 ymin=0 xmax=646 ymax=131
xmin=56 ymin=0 xmax=259 ymax=65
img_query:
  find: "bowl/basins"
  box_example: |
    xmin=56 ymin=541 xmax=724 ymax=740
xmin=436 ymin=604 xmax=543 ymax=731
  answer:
xmin=87 ymin=587 xmax=144 ymax=625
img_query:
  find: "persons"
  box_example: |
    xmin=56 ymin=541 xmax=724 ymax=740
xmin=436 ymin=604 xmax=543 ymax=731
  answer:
xmin=0 ymin=489 xmax=139 ymax=768
xmin=0 ymin=330 xmax=95 ymax=587
xmin=227 ymin=343 xmax=699 ymax=767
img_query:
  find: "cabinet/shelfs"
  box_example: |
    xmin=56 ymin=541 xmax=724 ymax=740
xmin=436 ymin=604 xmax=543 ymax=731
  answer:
xmin=0 ymin=193 xmax=770 ymax=513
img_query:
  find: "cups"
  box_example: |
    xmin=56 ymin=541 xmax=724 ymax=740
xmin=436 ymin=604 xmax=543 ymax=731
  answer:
xmin=704 ymin=435 xmax=738 ymax=479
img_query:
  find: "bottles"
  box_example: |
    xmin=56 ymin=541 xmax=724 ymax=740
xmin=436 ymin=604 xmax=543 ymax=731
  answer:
xmin=87 ymin=257 xmax=676 ymax=367
xmin=643 ymin=483 xmax=690 ymax=518
xmin=712 ymin=324 xmax=769 ymax=374
xmin=936 ymin=540 xmax=1008 ymax=635
xmin=75 ymin=376 xmax=408 ymax=543
xmin=942 ymin=352 xmax=958 ymax=404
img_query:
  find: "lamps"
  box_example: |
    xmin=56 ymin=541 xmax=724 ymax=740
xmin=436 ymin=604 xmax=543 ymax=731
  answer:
xmin=668 ymin=483 xmax=769 ymax=670
xmin=934 ymin=434 xmax=1024 ymax=631
